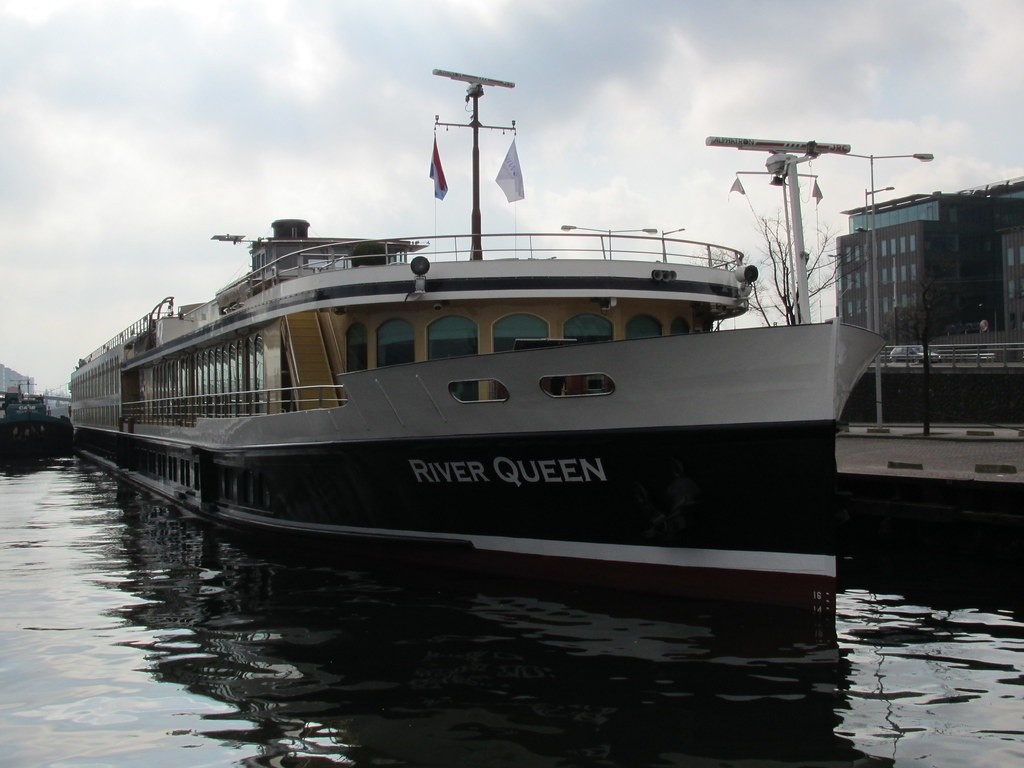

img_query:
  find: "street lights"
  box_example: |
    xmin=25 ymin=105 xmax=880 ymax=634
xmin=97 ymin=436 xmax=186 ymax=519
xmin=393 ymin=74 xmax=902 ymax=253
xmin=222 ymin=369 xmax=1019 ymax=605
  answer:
xmin=560 ymin=223 xmax=658 ymax=260
xmin=765 ymin=153 xmax=811 ymax=324
xmin=659 ymin=227 xmax=686 ymax=262
xmin=828 ymin=253 xmax=844 ymax=318
xmin=845 ymin=151 xmax=936 ymax=429
xmin=855 ymin=184 xmax=895 ymax=331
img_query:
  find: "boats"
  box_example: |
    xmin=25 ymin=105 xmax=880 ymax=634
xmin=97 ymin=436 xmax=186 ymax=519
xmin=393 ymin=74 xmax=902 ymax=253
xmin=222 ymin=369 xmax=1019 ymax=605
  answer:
xmin=0 ymin=377 xmax=74 ymax=461
xmin=66 ymin=67 xmax=890 ymax=594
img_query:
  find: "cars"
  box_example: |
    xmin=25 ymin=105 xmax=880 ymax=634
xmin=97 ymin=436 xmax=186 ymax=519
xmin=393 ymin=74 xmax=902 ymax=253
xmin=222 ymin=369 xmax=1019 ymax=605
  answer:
xmin=889 ymin=343 xmax=942 ymax=363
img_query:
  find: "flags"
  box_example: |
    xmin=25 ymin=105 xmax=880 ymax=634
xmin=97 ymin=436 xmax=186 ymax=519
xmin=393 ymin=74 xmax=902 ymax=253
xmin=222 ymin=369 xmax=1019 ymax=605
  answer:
xmin=728 ymin=178 xmax=745 ymax=202
xmin=495 ymin=139 xmax=524 ymax=202
xmin=429 ymin=140 xmax=448 ymax=200
xmin=813 ymin=181 xmax=823 ymax=204
